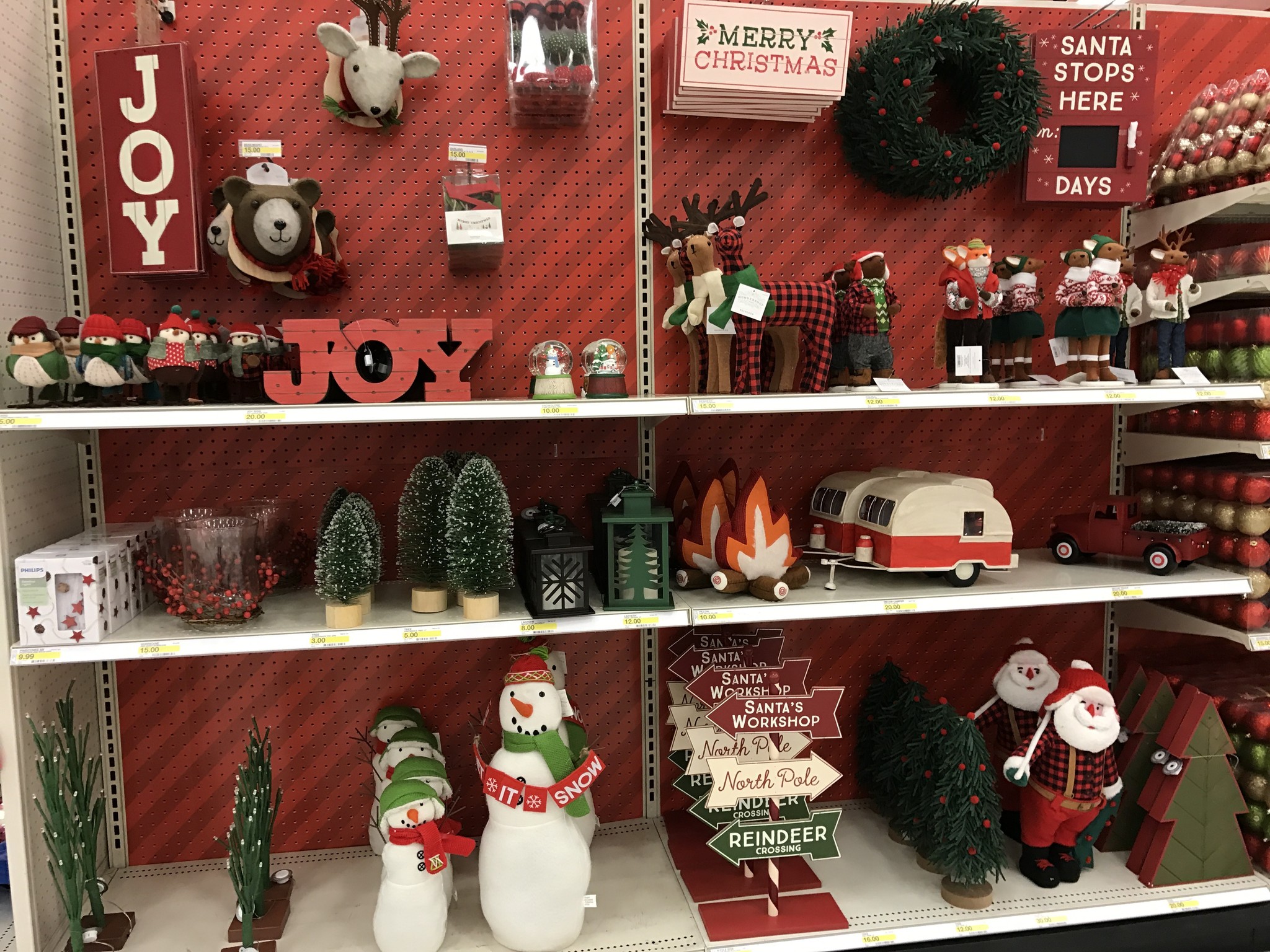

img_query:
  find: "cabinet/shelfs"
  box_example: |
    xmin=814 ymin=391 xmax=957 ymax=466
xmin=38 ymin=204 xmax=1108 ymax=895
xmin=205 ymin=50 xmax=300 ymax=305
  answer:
xmin=0 ymin=378 xmax=1270 ymax=950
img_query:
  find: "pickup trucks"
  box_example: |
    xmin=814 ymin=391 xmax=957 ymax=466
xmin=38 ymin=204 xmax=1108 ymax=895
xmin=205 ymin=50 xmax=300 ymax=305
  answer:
xmin=1047 ymin=496 xmax=1211 ymax=573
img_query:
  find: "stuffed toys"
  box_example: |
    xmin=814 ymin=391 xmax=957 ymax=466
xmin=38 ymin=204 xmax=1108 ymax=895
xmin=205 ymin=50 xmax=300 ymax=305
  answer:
xmin=939 ymin=232 xmax=1143 ymax=385
xmin=640 ymin=178 xmax=901 ymax=396
xmin=1145 ymin=223 xmax=1202 ymax=379
xmin=4 ymin=307 xmax=289 ymax=404
xmin=206 ymin=176 xmax=349 ymax=298
xmin=350 ymin=703 xmax=476 ymax=952
xmin=1001 ymin=659 xmax=1122 ymax=889
xmin=974 ymin=635 xmax=1061 ymax=844
xmin=315 ymin=0 xmax=441 ymax=130
xmin=470 ymin=644 xmax=597 ymax=951
